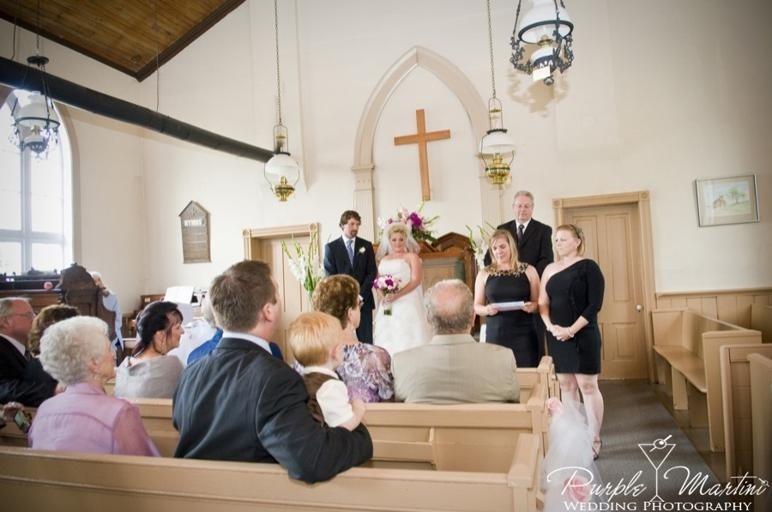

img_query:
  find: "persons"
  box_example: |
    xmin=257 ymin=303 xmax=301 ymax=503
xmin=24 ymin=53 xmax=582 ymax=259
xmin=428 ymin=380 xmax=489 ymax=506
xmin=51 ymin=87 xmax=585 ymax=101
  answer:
xmin=323 ymin=211 xmax=377 ymax=344
xmin=372 ymin=221 xmax=434 ymax=355
xmin=538 ymin=223 xmax=605 ymax=462
xmin=483 ymin=190 xmax=554 ymax=356
xmin=390 ymin=279 xmax=521 ymax=406
xmin=473 ymin=230 xmax=541 ymax=366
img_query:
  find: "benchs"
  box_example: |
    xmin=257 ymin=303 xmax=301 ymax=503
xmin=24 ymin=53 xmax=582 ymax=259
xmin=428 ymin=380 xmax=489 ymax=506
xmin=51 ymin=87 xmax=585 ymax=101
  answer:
xmin=650 ymin=298 xmax=772 ymax=510
xmin=1 ymin=351 xmax=562 ymax=512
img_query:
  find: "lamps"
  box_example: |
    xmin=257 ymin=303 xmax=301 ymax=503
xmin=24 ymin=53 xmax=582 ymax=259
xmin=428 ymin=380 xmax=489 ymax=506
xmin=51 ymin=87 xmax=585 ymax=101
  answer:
xmin=6 ymin=0 xmax=62 ymax=165
xmin=475 ymin=1 xmax=516 ymax=192
xmin=263 ymin=1 xmax=301 ymax=204
xmin=507 ymin=0 xmax=577 ymax=87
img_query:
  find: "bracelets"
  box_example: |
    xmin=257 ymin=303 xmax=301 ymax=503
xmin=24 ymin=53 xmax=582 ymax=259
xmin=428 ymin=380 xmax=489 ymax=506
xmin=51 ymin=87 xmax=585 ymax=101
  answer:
xmin=566 ymin=323 xmax=575 ymax=339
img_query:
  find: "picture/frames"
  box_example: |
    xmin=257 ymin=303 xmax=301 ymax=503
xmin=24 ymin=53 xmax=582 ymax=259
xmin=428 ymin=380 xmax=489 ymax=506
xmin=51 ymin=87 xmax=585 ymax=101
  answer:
xmin=696 ymin=168 xmax=761 ymax=229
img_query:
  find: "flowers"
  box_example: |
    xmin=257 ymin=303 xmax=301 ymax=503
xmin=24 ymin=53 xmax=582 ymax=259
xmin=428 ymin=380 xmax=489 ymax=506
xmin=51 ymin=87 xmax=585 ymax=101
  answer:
xmin=465 ymin=220 xmax=497 ymax=271
xmin=372 ymin=272 xmax=402 ymax=317
xmin=373 ymin=201 xmax=442 ymax=256
xmin=276 ymin=222 xmax=338 ymax=313
xmin=564 ymin=476 xmax=592 ymax=505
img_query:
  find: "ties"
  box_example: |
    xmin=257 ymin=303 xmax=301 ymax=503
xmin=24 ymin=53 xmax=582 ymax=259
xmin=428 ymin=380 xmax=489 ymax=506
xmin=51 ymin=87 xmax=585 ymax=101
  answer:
xmin=347 ymin=240 xmax=353 ymax=264
xmin=517 ymin=225 xmax=524 ymax=246
